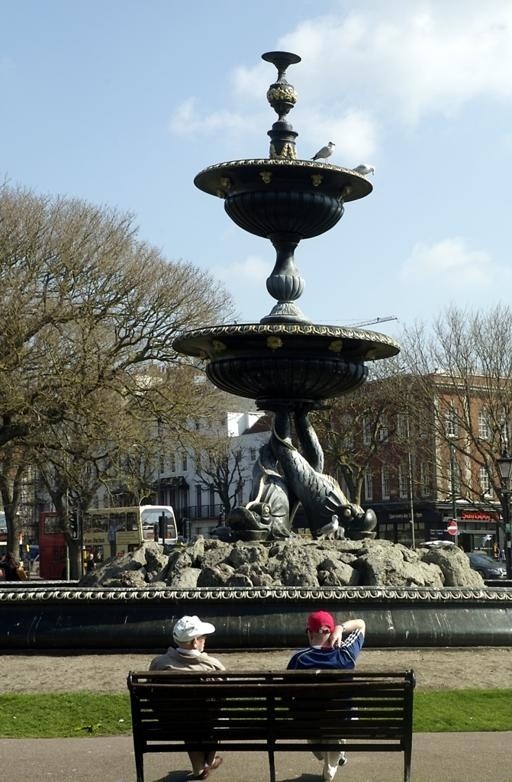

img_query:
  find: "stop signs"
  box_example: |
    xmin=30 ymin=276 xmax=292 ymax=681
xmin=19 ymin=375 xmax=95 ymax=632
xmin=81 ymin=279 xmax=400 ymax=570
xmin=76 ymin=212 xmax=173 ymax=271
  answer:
xmin=446 ymin=520 xmax=458 ymax=536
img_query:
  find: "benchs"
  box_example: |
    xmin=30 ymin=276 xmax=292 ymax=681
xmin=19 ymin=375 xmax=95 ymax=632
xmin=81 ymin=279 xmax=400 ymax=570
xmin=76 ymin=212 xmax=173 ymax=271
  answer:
xmin=126 ymin=670 xmax=416 ymax=782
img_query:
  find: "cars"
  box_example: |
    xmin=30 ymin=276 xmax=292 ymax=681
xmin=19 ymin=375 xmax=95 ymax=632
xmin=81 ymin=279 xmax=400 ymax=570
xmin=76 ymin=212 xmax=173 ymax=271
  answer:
xmin=467 ymin=551 xmax=506 ymax=580
xmin=1 ymin=543 xmax=39 ymax=565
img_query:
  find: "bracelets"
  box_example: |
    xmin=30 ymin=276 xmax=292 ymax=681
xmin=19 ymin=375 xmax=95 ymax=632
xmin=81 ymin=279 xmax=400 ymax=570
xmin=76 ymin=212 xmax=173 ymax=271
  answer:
xmin=339 ymin=624 xmax=347 ymax=634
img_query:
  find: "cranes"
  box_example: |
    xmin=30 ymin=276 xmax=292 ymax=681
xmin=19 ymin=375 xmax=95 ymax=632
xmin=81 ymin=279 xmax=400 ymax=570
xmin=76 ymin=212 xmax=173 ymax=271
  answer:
xmin=343 ymin=314 xmax=398 ymax=328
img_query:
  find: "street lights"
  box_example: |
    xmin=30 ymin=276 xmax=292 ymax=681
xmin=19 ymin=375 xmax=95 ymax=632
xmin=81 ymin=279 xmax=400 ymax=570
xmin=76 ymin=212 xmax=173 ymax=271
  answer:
xmin=496 ymin=443 xmax=511 ymax=588
xmin=447 ymin=432 xmax=458 ymax=546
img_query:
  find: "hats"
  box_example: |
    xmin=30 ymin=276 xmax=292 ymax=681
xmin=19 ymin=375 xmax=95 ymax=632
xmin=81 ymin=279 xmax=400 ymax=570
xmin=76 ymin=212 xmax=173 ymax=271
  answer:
xmin=305 ymin=610 xmax=335 ymax=635
xmin=172 ymin=615 xmax=216 ymax=644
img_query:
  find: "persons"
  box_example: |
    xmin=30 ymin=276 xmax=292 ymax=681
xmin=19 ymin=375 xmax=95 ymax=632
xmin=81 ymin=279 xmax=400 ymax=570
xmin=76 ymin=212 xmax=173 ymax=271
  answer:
xmin=280 ymin=609 xmax=367 ymax=782
xmin=86 ymin=553 xmax=96 ymax=574
xmin=148 ymin=614 xmax=229 ymax=781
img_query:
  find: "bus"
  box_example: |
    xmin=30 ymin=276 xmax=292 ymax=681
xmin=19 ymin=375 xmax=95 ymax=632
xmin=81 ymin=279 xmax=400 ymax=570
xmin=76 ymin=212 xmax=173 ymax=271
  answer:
xmin=38 ymin=505 xmax=177 ymax=580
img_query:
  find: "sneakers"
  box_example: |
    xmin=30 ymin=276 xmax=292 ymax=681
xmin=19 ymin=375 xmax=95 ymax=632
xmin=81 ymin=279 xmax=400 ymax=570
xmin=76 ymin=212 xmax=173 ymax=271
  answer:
xmin=338 ymin=756 xmax=348 ymax=768
xmin=210 ymin=756 xmax=223 ymax=769
xmin=194 ymin=764 xmax=209 ymax=779
